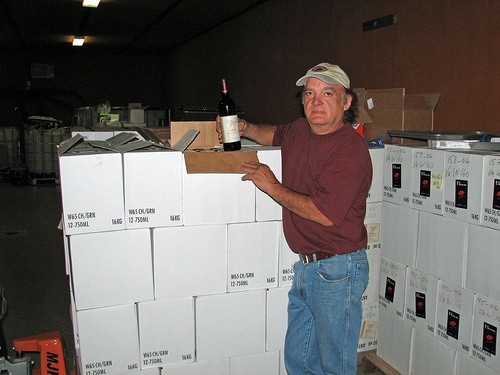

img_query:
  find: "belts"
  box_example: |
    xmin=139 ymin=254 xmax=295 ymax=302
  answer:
xmin=299 ymin=252 xmax=333 ymax=266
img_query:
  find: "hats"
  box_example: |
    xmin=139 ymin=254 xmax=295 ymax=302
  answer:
xmin=296 ymin=62 xmax=350 ymax=89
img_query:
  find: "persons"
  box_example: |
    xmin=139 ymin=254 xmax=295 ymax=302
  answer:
xmin=216 ymin=63 xmax=373 ymax=375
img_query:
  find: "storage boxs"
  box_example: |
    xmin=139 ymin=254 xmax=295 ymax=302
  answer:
xmin=56 ymin=88 xmax=500 ymax=375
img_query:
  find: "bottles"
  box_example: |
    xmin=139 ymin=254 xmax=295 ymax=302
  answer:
xmin=218 ymin=79 xmax=241 ymax=151
